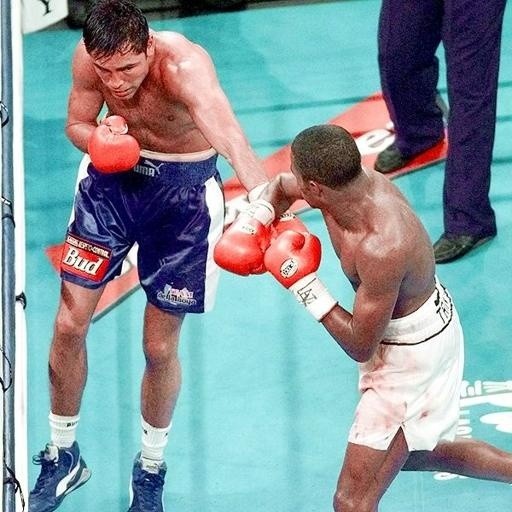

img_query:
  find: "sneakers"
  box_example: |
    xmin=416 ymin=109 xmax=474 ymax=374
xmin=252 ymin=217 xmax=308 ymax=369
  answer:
xmin=124 ymin=450 xmax=167 ymax=512
xmin=28 ymin=441 xmax=91 ymax=511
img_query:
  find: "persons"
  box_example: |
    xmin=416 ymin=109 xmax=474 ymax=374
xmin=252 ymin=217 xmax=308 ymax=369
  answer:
xmin=375 ymin=2 xmax=507 ymax=262
xmin=25 ymin=0 xmax=290 ymax=512
xmin=215 ymin=120 xmax=512 ymax=510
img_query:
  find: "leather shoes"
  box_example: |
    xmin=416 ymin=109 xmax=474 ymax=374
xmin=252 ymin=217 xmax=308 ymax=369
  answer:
xmin=432 ymin=231 xmax=496 ymax=264
xmin=374 ymin=142 xmax=403 ymax=174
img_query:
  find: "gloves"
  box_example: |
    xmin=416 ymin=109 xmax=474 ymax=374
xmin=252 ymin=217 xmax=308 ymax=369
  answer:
xmin=213 ymin=198 xmax=276 ymax=278
xmin=247 ymin=183 xmax=309 ymax=249
xmin=87 ymin=115 xmax=140 ymax=174
xmin=263 ymin=229 xmax=339 ymax=323
